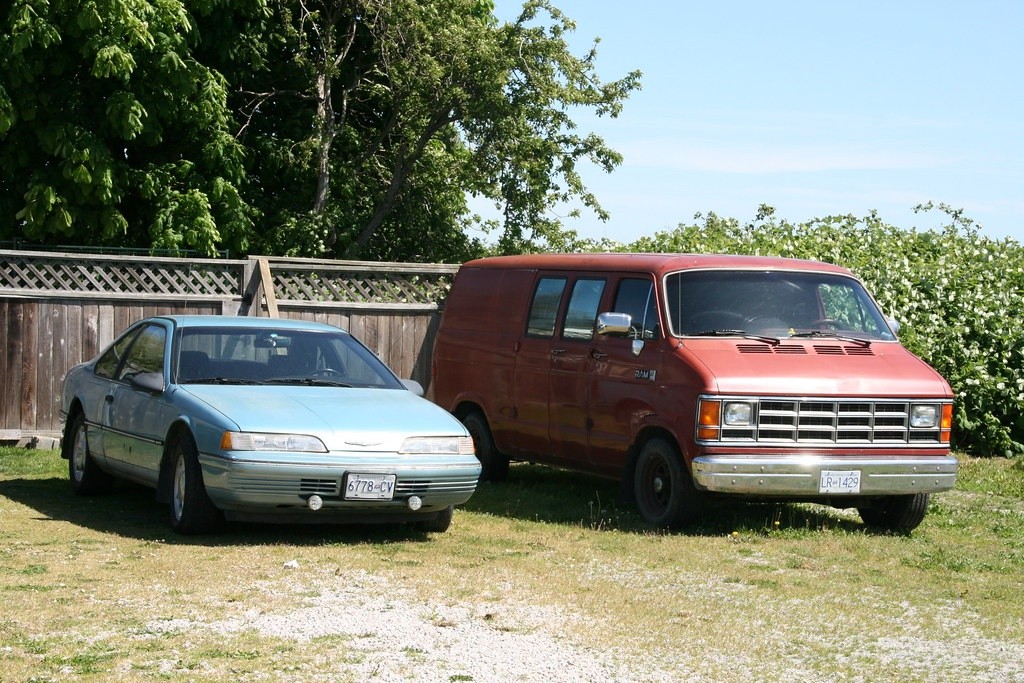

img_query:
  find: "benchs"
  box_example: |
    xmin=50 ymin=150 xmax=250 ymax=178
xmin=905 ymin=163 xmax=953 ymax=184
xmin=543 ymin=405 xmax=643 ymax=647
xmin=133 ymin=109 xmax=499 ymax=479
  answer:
xmin=210 ymin=358 xmax=267 ymax=380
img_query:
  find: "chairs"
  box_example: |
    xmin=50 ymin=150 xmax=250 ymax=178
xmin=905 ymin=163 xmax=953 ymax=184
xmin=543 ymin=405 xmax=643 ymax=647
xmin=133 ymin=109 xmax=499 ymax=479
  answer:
xmin=264 ymin=355 xmax=300 ymax=378
xmin=180 ymin=352 xmax=213 ymax=380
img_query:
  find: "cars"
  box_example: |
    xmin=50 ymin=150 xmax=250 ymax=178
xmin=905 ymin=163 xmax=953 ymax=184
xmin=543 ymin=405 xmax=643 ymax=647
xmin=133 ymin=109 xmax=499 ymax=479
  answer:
xmin=58 ymin=315 xmax=483 ymax=533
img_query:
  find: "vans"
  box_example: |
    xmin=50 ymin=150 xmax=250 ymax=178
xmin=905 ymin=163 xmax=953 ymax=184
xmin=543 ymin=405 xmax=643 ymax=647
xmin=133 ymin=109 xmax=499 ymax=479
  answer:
xmin=432 ymin=251 xmax=957 ymax=533
